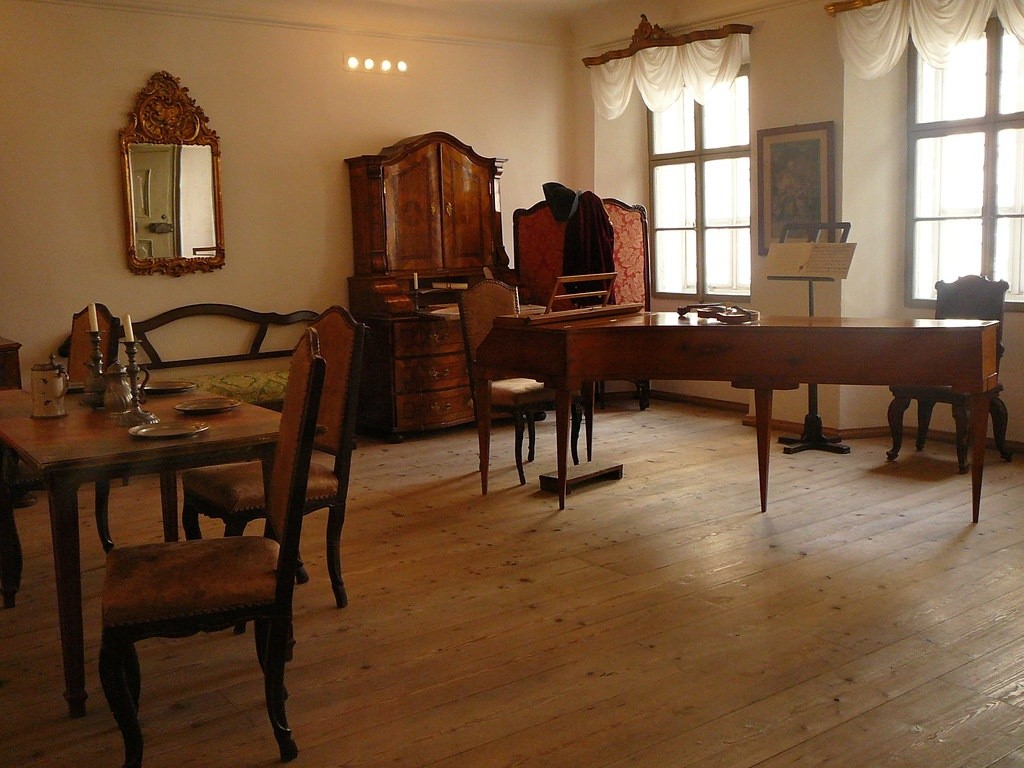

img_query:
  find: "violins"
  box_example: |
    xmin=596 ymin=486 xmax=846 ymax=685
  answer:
xmin=676 ymin=294 xmax=761 ymax=324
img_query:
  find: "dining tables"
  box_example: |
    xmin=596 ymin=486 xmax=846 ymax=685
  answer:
xmin=0 ymin=379 xmax=328 ymax=720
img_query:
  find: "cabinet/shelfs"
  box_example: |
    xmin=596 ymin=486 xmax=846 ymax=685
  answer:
xmin=344 ymin=131 xmax=520 ymax=439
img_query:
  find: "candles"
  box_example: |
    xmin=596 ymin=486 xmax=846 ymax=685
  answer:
xmin=88 ymin=301 xmax=99 ymax=331
xmin=414 ymin=271 xmax=419 ymax=289
xmin=121 ymin=310 xmax=134 ymax=341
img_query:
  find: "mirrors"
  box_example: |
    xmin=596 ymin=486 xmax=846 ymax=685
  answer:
xmin=118 ymin=70 xmax=226 ymax=277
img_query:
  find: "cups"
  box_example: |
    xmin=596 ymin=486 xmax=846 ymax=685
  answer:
xmin=30 ymin=362 xmax=70 ymax=419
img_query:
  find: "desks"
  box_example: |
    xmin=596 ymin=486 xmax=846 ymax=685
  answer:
xmin=494 ymin=312 xmax=1002 ymax=524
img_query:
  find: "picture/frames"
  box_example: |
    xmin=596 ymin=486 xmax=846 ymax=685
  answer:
xmin=757 ymin=119 xmax=837 ymax=257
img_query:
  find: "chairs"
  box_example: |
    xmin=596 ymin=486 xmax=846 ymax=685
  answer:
xmin=181 ymin=303 xmax=368 ymax=635
xmin=459 ymin=277 xmax=584 ymax=486
xmin=99 ymin=324 xmax=329 ymax=768
xmin=885 ymin=274 xmax=1013 ymax=473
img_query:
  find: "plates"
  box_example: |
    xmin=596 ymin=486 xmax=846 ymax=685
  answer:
xmin=127 ymin=419 xmax=210 ymax=440
xmin=137 ymin=380 xmax=197 ymax=394
xmin=172 ymin=397 xmax=242 ymax=413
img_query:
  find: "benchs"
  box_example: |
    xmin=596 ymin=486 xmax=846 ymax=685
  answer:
xmin=118 ymin=305 xmax=320 ymax=413
